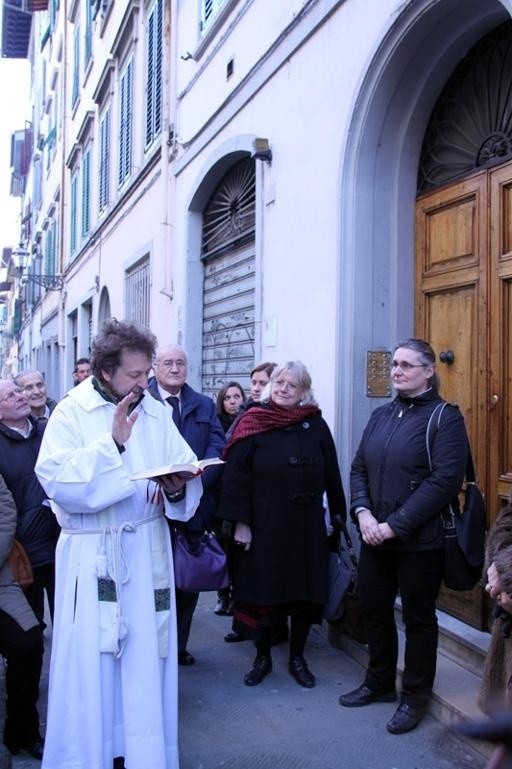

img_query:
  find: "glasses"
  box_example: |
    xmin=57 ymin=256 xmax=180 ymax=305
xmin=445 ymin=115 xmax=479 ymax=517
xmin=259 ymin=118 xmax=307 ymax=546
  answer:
xmin=390 ymin=361 xmax=429 ymax=371
xmin=0 ymin=386 xmax=25 ymax=404
xmin=272 ymin=378 xmax=304 ymax=392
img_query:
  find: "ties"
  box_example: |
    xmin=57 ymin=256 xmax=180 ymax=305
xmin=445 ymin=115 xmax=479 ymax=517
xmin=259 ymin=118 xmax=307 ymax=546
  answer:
xmin=165 ymin=397 xmax=180 ymax=432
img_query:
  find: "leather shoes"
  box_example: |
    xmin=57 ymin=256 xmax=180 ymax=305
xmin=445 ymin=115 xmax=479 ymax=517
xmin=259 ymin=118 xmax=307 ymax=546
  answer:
xmin=387 ymin=696 xmax=427 ymax=734
xmin=179 ymin=650 xmax=194 ymax=665
xmin=254 ymin=632 xmax=289 ymax=648
xmin=225 ymin=631 xmax=247 ymax=642
xmin=289 ymin=657 xmax=315 ymax=688
xmin=339 ymin=682 xmax=398 ymax=707
xmin=243 ymin=654 xmax=272 ymax=685
xmin=214 ymin=594 xmax=230 ymax=615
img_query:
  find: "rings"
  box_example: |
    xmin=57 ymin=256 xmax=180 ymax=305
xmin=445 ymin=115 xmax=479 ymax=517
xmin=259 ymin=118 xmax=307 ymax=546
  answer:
xmin=489 ymin=584 xmax=493 ymax=589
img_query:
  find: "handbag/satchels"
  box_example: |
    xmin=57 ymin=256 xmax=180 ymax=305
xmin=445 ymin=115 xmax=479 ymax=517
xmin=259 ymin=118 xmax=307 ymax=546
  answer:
xmin=442 ymin=482 xmax=488 ymax=592
xmin=172 ymin=527 xmax=232 ymax=593
xmin=7 ymin=539 xmax=34 ymax=593
xmin=322 ymin=531 xmax=358 ymax=624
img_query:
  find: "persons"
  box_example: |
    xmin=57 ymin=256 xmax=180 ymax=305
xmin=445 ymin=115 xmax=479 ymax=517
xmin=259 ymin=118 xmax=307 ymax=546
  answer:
xmin=72 ymin=359 xmax=91 ymax=386
xmin=146 ymin=345 xmax=227 ymax=664
xmin=1 ymin=475 xmax=44 ymax=760
xmin=14 ymin=368 xmax=59 ymax=430
xmin=224 ymin=360 xmax=345 ymax=687
xmin=213 ymin=381 xmax=248 ymax=615
xmin=1 ymin=381 xmax=61 ymax=630
xmin=225 ymin=362 xmax=289 ymax=647
xmin=476 ymin=499 xmax=512 ymax=725
xmin=33 ymin=316 xmax=207 ymax=769
xmin=339 ymin=337 xmax=469 ymax=732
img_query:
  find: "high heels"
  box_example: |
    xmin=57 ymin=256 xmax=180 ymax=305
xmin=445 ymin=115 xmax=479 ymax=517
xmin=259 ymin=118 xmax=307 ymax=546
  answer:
xmin=1 ymin=732 xmax=45 ymax=761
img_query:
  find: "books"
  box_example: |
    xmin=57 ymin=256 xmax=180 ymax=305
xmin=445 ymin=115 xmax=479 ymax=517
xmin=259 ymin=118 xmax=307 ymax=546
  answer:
xmin=129 ymin=455 xmax=226 ymax=479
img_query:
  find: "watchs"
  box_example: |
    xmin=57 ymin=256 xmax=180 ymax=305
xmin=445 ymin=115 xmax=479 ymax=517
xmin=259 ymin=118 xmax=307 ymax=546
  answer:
xmin=169 ymin=488 xmax=185 ymax=500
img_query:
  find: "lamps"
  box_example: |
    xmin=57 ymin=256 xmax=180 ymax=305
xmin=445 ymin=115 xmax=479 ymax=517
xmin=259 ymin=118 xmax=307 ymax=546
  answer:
xmin=10 ymin=242 xmax=65 ymax=292
